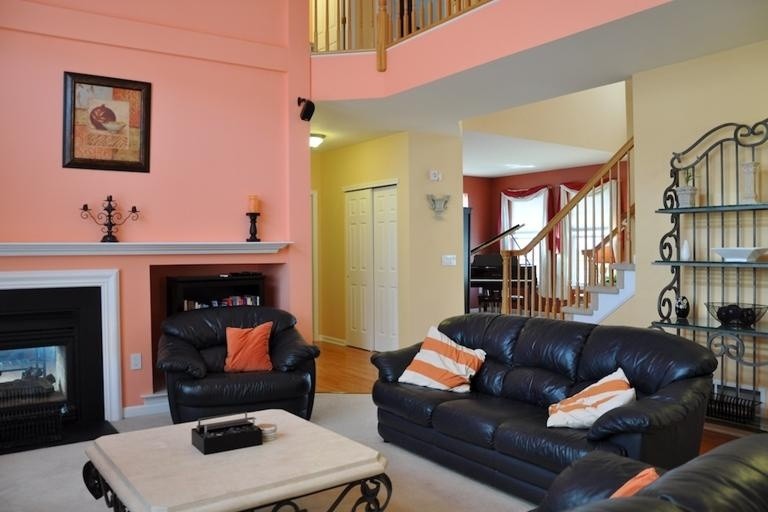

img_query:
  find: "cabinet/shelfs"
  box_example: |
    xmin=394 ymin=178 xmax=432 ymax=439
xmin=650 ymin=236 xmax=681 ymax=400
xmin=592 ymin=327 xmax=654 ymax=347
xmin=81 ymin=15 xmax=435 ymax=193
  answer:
xmin=165 ymin=272 xmax=267 ymax=317
xmin=648 ymin=112 xmax=767 ymax=432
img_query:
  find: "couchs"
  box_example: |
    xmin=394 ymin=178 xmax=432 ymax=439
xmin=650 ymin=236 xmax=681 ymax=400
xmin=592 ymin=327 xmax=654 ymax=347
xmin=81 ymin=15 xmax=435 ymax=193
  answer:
xmin=368 ymin=309 xmax=718 ymax=507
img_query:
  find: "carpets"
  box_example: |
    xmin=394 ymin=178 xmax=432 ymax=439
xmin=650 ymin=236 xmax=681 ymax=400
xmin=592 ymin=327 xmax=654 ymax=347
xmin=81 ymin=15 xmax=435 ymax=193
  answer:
xmin=0 ymin=391 xmax=540 ymax=512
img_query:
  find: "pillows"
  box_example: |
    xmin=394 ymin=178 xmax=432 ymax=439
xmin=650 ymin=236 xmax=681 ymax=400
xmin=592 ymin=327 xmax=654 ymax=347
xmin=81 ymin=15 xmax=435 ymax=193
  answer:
xmin=603 ymin=463 xmax=661 ymax=502
xmin=395 ymin=324 xmax=488 ymax=395
xmin=544 ymin=365 xmax=637 ymax=431
xmin=219 ymin=314 xmax=275 ymax=374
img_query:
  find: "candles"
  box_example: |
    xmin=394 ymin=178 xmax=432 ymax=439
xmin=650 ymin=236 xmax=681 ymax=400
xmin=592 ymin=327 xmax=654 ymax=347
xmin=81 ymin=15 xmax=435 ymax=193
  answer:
xmin=248 ymin=194 xmax=260 ymax=213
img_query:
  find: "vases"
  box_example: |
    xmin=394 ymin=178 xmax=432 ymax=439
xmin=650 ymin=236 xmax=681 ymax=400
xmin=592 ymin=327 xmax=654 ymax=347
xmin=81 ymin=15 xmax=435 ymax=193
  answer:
xmin=679 ymin=239 xmax=691 ymax=261
xmin=739 ymin=160 xmax=760 ymax=205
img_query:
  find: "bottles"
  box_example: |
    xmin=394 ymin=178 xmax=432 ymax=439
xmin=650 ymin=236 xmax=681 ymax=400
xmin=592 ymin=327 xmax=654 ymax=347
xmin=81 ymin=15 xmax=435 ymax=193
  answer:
xmin=681 ymin=240 xmax=691 ymax=261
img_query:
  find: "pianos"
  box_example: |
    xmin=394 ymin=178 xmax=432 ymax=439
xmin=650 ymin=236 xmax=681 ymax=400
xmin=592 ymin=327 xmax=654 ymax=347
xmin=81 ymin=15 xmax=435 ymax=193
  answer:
xmin=471 ymin=224 xmax=537 ymax=315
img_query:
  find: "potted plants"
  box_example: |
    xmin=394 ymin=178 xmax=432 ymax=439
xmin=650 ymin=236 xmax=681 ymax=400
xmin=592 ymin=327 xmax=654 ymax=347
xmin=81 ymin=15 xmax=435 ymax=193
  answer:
xmin=672 ymin=166 xmax=699 ymax=209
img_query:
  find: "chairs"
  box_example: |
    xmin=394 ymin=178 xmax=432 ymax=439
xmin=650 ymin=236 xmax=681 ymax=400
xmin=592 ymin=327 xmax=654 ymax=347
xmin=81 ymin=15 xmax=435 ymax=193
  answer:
xmin=528 ymin=428 xmax=766 ymax=512
xmin=154 ymin=301 xmax=322 ymax=426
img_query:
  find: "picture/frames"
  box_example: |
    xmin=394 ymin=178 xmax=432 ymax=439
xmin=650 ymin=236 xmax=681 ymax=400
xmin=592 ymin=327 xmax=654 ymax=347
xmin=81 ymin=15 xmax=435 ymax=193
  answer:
xmin=61 ymin=68 xmax=153 ymax=173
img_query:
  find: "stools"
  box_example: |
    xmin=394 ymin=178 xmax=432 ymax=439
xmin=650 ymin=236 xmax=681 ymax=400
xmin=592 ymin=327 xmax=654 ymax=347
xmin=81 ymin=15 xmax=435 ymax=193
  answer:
xmin=477 ymin=294 xmax=525 ymax=313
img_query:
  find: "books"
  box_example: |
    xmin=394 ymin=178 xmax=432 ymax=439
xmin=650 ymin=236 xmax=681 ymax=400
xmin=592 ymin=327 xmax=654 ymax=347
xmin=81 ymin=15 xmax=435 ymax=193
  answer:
xmin=177 ymin=294 xmax=260 ymax=311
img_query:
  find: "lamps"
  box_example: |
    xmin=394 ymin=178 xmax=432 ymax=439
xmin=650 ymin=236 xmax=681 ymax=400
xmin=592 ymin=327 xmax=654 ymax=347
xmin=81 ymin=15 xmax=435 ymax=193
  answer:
xmin=309 ymin=132 xmax=327 ymax=150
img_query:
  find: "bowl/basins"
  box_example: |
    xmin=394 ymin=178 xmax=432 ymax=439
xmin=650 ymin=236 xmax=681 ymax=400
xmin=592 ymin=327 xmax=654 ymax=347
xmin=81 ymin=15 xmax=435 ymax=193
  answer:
xmin=710 ymin=247 xmax=766 ymax=263
